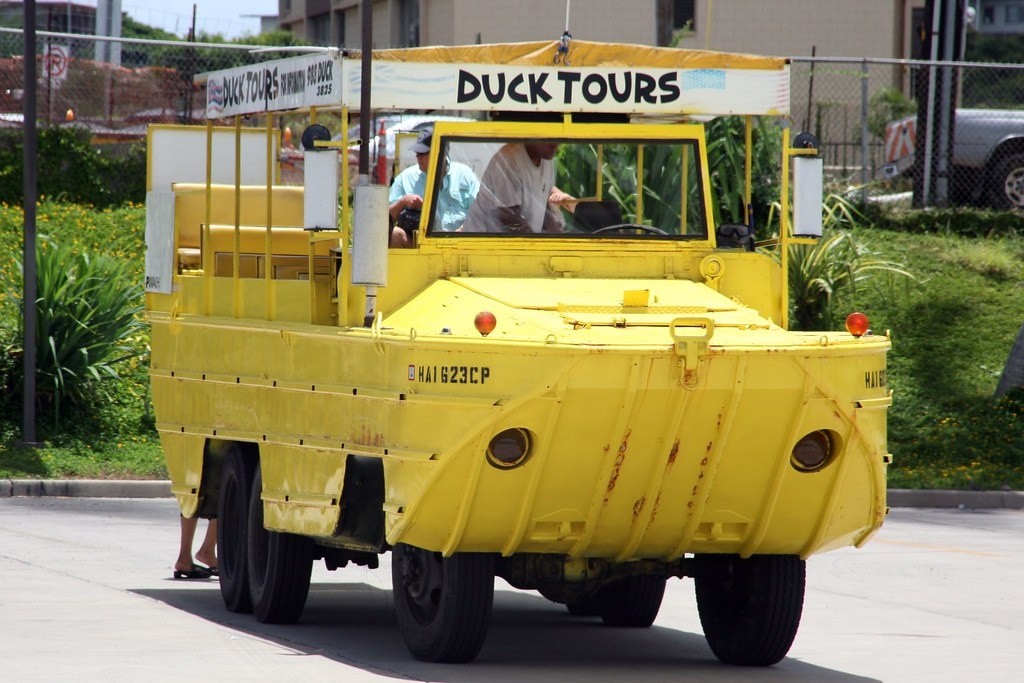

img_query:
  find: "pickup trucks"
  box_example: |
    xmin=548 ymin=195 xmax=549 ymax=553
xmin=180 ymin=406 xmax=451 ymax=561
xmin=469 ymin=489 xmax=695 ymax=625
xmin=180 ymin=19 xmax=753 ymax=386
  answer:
xmin=884 ymin=108 xmax=1023 ymax=211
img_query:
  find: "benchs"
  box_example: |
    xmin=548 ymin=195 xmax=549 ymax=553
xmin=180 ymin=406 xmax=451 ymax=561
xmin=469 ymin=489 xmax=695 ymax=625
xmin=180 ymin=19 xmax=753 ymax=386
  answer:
xmin=172 ymin=183 xmax=340 ymax=280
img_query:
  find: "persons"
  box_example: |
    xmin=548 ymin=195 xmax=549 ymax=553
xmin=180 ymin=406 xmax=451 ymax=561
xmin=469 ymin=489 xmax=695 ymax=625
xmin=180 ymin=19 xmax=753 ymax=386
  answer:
xmin=388 ymin=126 xmax=479 ymax=248
xmin=463 ymin=137 xmax=575 ymax=232
xmin=173 ymin=513 xmax=220 ymax=579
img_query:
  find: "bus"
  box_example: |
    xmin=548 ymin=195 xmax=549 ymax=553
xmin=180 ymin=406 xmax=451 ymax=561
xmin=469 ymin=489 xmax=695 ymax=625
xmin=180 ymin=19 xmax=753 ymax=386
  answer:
xmin=144 ymin=30 xmax=894 ymax=667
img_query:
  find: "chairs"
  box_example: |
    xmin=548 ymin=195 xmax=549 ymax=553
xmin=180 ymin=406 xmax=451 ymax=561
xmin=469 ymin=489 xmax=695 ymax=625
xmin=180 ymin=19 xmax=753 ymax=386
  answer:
xmin=573 ymin=201 xmax=622 ymax=233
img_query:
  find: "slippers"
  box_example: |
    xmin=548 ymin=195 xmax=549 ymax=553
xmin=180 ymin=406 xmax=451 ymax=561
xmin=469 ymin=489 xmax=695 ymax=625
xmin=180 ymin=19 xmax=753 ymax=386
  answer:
xmin=174 ymin=564 xmax=211 ymax=580
xmin=202 ymin=561 xmax=221 ymax=576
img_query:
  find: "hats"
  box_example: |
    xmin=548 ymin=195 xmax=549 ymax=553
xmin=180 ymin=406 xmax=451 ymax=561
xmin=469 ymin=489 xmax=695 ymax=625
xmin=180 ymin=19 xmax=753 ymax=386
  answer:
xmin=411 ymin=127 xmax=434 ymax=153
